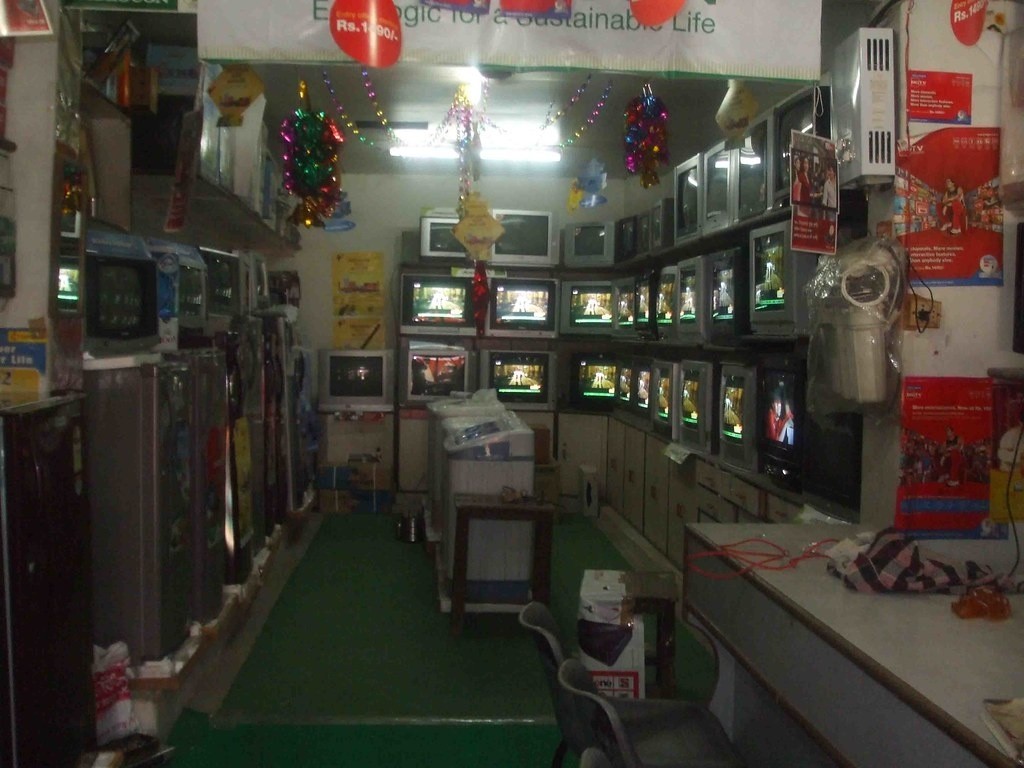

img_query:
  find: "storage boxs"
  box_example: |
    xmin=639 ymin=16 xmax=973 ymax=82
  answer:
xmin=527 ymin=423 xmax=552 ymax=465
xmin=534 ymin=458 xmax=563 ymax=525
xmin=315 ymin=467 xmax=347 ymax=490
xmin=347 ymin=456 xmax=394 ymax=492
xmin=368 ymin=490 xmax=392 ymax=515
xmin=317 ymin=488 xmax=370 ymax=514
xmin=578 ymin=464 xmax=599 ymax=519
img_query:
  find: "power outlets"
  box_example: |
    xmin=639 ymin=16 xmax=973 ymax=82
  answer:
xmin=908 ymin=300 xmax=941 ymax=325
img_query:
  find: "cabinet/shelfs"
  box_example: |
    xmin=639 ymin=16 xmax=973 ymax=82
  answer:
xmin=399 ymin=192 xmax=888 ymax=571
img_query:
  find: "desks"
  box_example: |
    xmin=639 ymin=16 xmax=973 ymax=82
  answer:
xmin=676 ymin=522 xmax=1024 ymax=768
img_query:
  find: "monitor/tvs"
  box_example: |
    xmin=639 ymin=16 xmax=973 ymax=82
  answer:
xmin=399 ymin=86 xmax=837 ymax=486
xmin=316 ymin=349 xmax=395 ymax=413
xmin=84 ymin=244 xmax=246 ymax=371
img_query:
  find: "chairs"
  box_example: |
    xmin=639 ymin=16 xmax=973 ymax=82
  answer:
xmin=556 ymin=658 xmax=645 ymax=768
xmin=516 ymin=601 xmax=746 ymax=768
